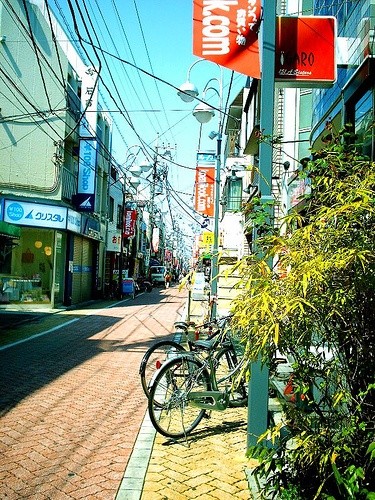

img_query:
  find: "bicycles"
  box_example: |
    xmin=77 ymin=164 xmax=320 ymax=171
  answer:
xmin=139 ymin=314 xmax=238 ymax=411
xmin=147 ymin=313 xmax=253 ymax=448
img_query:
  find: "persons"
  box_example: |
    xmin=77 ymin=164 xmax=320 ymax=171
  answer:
xmin=162 ymin=268 xmax=192 ymax=288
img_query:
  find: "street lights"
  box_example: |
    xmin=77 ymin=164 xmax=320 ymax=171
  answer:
xmin=178 ymin=57 xmax=224 ymax=328
xmin=116 ymin=145 xmax=153 ymax=300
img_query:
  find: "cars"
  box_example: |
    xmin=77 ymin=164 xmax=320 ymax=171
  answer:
xmin=252 ymin=312 xmax=355 ymax=412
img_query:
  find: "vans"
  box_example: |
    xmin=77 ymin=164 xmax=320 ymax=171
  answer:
xmin=148 ymin=266 xmax=167 ymax=286
